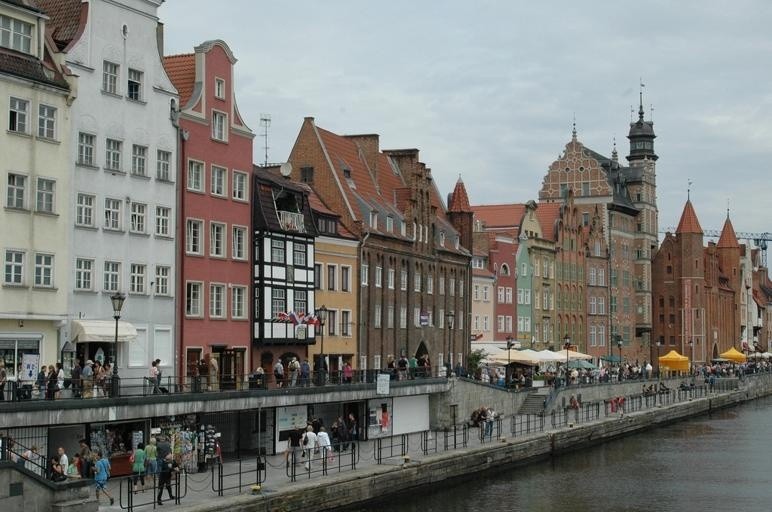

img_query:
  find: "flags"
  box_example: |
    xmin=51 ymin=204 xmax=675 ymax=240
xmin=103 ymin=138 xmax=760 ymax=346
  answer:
xmin=279 ymin=311 xmax=321 ymax=326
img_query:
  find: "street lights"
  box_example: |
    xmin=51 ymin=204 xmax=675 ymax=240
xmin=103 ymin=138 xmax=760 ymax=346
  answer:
xmin=616 ymin=337 xmax=626 ymax=382
xmin=754 ymin=341 xmax=759 ymax=374
xmin=316 ymin=304 xmax=330 ymax=384
xmin=108 ymin=291 xmax=126 ymax=397
xmin=562 ymin=333 xmax=572 ymax=386
xmin=653 ymin=336 xmax=663 ymax=378
xmin=688 ymin=340 xmax=694 ymax=376
xmin=502 ymin=335 xmax=514 ymax=388
xmin=445 ymin=311 xmax=453 ymax=376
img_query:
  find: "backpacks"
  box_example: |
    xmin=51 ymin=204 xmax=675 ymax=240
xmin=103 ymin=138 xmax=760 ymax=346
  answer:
xmin=290 ymin=361 xmax=297 ymax=371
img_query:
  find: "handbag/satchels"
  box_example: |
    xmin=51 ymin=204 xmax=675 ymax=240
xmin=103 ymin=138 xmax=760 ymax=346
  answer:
xmin=303 ymin=432 xmax=309 ymax=445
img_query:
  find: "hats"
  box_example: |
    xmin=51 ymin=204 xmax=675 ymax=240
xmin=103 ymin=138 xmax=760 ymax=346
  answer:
xmin=86 ymin=360 xmax=94 ymax=365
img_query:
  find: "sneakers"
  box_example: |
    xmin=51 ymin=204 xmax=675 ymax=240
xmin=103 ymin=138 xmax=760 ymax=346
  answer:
xmin=109 ymin=497 xmax=115 ymax=505
xmin=135 ymin=490 xmax=176 ymax=505
xmin=304 ymin=465 xmax=309 ymax=471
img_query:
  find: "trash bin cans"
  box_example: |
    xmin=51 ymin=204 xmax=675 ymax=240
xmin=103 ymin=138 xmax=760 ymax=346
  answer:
xmin=249 ymin=373 xmax=263 ymax=390
xmin=23 ymin=384 xmax=32 ymax=399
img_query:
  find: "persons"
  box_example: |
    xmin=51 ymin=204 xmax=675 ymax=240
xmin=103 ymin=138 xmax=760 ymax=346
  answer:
xmin=0 ymin=353 xmax=429 ymax=512
xmin=471 ymin=357 xmax=772 ymax=441
